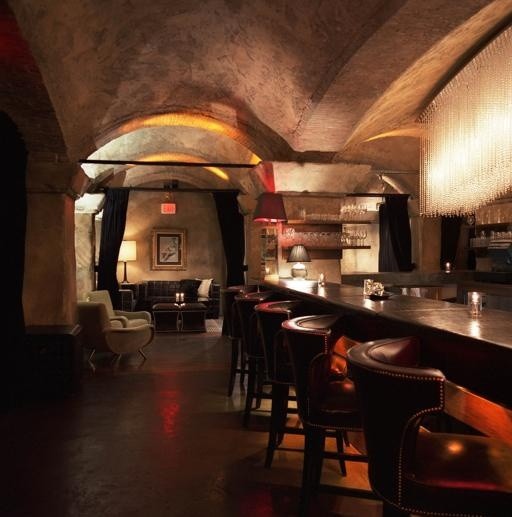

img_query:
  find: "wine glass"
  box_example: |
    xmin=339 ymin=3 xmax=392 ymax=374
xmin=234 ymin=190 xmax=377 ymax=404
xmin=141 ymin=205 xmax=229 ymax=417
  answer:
xmin=345 ymin=226 xmax=367 ymax=246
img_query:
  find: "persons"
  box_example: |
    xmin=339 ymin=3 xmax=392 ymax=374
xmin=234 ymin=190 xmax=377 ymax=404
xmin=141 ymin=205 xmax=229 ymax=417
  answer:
xmin=161 ymin=237 xmax=178 ymax=262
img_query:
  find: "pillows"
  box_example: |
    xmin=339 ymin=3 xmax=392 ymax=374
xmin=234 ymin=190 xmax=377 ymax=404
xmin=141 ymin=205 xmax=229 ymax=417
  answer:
xmin=178 ymin=277 xmax=214 ymax=302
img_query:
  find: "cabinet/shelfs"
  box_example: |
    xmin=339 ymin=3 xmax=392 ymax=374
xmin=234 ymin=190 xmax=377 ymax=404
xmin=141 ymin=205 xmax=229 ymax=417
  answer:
xmin=280 ymin=219 xmax=372 ymax=253
xmin=461 ymin=220 xmax=512 ymax=253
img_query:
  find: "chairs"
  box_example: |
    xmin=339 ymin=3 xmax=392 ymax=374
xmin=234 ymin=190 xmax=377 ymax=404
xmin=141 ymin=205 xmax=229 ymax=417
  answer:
xmin=77 ymin=288 xmax=155 ymax=370
xmin=253 ymin=300 xmax=351 ymax=475
xmin=347 ymin=334 xmax=511 ymax=517
xmin=281 ymin=313 xmax=391 ymax=515
xmin=236 ymin=288 xmax=288 ymax=419
xmin=220 ymin=285 xmax=259 ymax=398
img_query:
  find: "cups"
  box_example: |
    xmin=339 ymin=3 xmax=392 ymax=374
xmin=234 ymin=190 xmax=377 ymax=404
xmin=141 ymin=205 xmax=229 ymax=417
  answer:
xmin=363 ymin=278 xmax=373 ymax=295
xmin=467 ymin=292 xmax=482 ymax=317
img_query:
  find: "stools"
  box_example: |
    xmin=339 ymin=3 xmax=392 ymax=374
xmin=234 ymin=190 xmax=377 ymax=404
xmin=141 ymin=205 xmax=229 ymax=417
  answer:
xmin=151 ymin=301 xmax=209 ymax=335
xmin=114 ymin=282 xmax=139 ymax=313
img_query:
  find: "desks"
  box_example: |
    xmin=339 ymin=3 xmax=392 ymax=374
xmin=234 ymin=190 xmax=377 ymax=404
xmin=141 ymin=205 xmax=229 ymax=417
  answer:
xmin=260 ymin=270 xmax=510 ymax=434
xmin=262 ymin=283 xmax=510 ymax=407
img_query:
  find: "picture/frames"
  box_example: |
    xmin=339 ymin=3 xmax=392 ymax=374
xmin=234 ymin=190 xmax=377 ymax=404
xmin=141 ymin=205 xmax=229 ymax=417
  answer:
xmin=150 ymin=227 xmax=188 ymax=271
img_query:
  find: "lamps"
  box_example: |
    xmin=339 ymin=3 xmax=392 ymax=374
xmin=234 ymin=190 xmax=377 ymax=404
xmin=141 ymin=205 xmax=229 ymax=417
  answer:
xmin=411 ymin=10 xmax=512 ymax=220
xmin=160 ymin=177 xmax=177 ymax=214
xmin=287 ymin=243 xmax=312 ymax=279
xmin=117 ymin=240 xmax=137 ymax=283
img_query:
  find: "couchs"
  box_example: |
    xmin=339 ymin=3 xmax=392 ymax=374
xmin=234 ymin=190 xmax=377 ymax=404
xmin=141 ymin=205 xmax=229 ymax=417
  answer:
xmin=143 ymin=280 xmax=221 ymax=320
